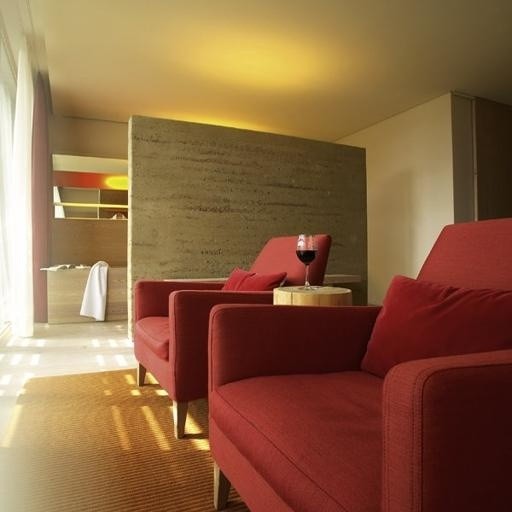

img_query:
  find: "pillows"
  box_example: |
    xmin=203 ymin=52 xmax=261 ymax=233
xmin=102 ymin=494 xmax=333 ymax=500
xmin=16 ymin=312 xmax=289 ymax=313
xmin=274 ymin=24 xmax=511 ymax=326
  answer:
xmin=359 ymin=274 xmax=512 ymax=377
xmin=221 ymin=265 xmax=287 ymax=293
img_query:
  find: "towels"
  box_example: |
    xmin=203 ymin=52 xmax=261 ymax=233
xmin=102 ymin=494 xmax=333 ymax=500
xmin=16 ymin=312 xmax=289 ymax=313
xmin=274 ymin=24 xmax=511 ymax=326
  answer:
xmin=79 ymin=260 xmax=109 ymax=322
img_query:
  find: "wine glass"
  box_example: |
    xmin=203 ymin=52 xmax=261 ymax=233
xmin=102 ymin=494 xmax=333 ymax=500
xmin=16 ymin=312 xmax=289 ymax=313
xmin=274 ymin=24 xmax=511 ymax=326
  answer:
xmin=295 ymin=232 xmax=319 ymax=290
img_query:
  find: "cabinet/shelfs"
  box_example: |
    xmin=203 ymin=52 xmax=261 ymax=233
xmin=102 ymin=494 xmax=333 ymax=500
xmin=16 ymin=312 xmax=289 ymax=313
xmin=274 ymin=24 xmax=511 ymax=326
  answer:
xmin=53 ymin=185 xmax=128 ymax=221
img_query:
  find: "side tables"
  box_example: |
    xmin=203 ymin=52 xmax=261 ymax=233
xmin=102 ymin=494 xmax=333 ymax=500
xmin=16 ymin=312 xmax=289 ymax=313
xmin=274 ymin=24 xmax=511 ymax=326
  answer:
xmin=272 ymin=285 xmax=354 ymax=307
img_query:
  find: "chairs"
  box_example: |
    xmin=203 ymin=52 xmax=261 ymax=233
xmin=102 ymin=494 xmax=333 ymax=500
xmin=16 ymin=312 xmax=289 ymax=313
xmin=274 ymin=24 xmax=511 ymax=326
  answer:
xmin=207 ymin=218 xmax=512 ymax=512
xmin=134 ymin=233 xmax=332 ymax=439
